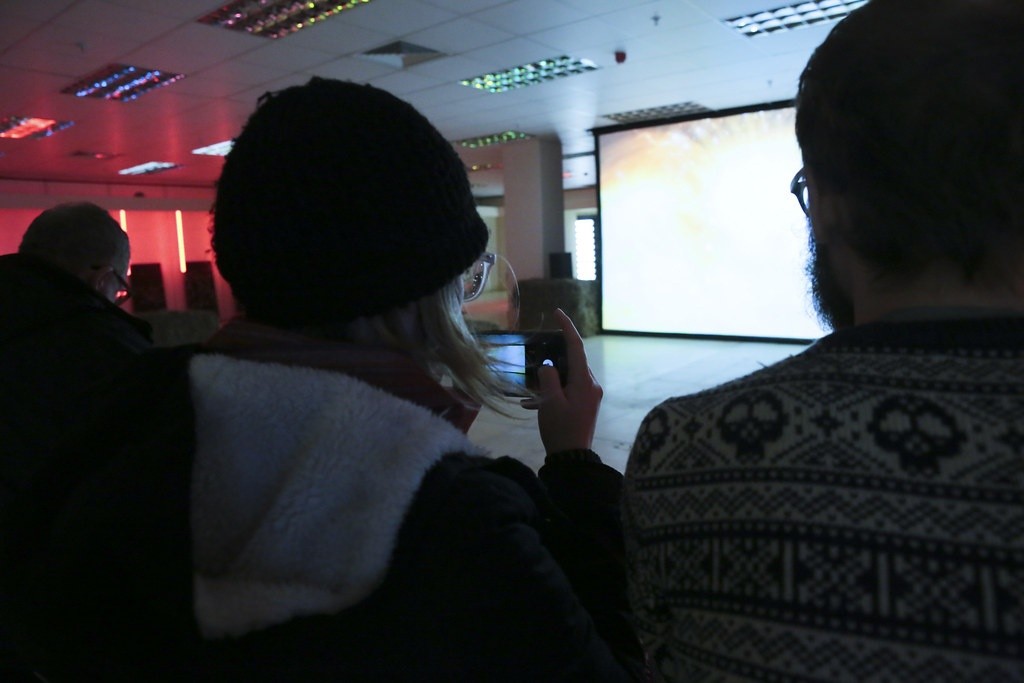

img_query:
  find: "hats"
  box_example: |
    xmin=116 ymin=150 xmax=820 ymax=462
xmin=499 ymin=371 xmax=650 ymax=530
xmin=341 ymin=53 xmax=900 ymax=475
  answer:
xmin=211 ymin=76 xmax=488 ymax=328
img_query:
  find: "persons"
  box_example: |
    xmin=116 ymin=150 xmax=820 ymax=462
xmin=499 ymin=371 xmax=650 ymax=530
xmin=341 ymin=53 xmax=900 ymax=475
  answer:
xmin=0 ymin=201 xmax=154 ymax=484
xmin=23 ymin=77 xmax=646 ymax=683
xmin=619 ymin=1 xmax=1024 ymax=683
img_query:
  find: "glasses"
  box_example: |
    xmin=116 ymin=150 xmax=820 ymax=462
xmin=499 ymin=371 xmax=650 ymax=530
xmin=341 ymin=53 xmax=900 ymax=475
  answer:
xmin=92 ymin=264 xmax=132 ymax=306
xmin=461 ymin=251 xmax=496 ymax=302
xmin=791 ymin=165 xmax=839 ymax=217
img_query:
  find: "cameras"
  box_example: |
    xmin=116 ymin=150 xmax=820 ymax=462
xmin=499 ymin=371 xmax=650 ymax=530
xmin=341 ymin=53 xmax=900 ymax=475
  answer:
xmin=471 ymin=330 xmax=568 ymax=398
